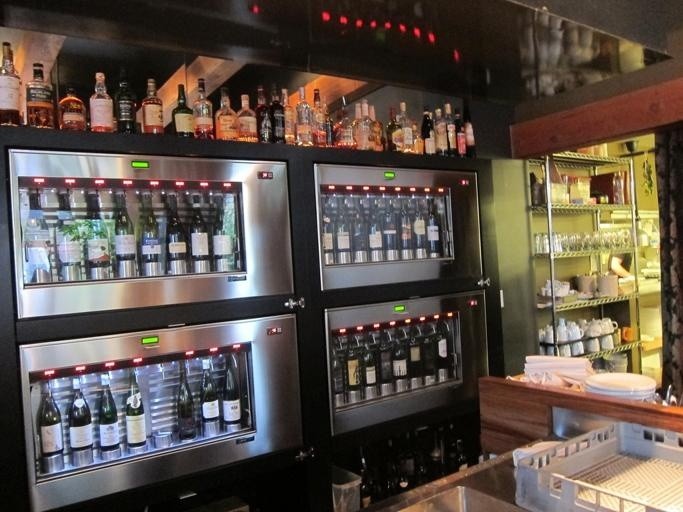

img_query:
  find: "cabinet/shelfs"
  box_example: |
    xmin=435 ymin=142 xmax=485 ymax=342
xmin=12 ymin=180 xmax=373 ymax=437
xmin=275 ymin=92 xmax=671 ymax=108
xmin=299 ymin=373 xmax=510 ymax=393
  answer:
xmin=524 ymin=150 xmax=642 ymax=380
xmin=0 ymin=126 xmax=493 ymax=512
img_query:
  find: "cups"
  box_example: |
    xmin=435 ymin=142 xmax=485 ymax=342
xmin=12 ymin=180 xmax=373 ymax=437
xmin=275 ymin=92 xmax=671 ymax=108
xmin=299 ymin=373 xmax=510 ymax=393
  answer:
xmin=537 ymin=317 xmax=621 ymax=357
xmin=535 ymin=230 xmax=632 ymax=254
xmin=622 ymin=326 xmax=635 ymax=343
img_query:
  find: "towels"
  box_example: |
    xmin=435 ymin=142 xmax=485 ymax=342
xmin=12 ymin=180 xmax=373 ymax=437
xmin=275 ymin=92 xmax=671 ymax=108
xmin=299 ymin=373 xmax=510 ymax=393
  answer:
xmin=522 ymin=354 xmax=593 ymax=392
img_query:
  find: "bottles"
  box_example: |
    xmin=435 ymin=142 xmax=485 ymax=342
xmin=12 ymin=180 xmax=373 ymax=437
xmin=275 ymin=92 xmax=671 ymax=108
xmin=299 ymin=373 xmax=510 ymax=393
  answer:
xmin=363 ymin=331 xmax=376 ymax=386
xmin=87 ymin=194 xmax=109 ymax=266
xmin=237 ymin=95 xmax=257 ymax=142
xmin=281 ymin=89 xmax=296 ymax=144
xmin=321 ymin=197 xmax=333 ymax=253
xmin=69 ymin=389 xmax=93 ymax=451
xmin=368 ymin=199 xmax=381 ymax=248
xmin=421 ymin=106 xmax=436 ymax=153
xmin=427 ymin=199 xmax=441 ymax=253
xmin=189 ymin=196 xmax=210 ymax=259
xmin=332 ymin=347 xmax=344 ymax=394
xmin=172 ymin=84 xmax=193 ymax=138
xmin=26 ymin=62 xmax=55 ymax=130
xmin=297 ymin=87 xmax=313 ymax=146
xmin=166 ymin=194 xmax=185 ymax=259
xmin=409 ymin=324 xmax=422 ymax=378
xmin=268 ymin=83 xmax=286 ymax=143
xmin=326 ymin=94 xmax=383 ymax=151
xmin=199 ymin=356 xmax=219 ymax=420
xmin=422 ymin=322 xmax=435 ymax=375
xmin=345 ymin=333 xmax=361 ymax=390
xmin=215 ymin=87 xmax=237 ymax=140
xmin=437 ymin=323 xmax=448 ymax=369
xmin=412 ymin=199 xmax=425 ymax=247
xmin=60 ymin=191 xmax=82 ymax=264
xmin=39 ymin=390 xmax=64 ymax=457
xmin=0 ymin=42 xmax=22 ymax=125
xmin=255 ymin=85 xmax=272 ymax=142
xmin=378 ymin=331 xmax=394 ymax=383
xmin=399 ymin=197 xmax=412 ymax=249
xmin=413 ymin=123 xmax=423 ymax=154
xmin=383 ymin=198 xmax=397 ymax=249
xmin=222 ymin=353 xmax=240 ymax=422
xmin=126 ymin=371 xmax=145 ymax=447
xmin=142 ymin=194 xmax=159 ymax=262
xmin=396 ymin=102 xmax=413 ymax=151
xmin=390 ymin=328 xmax=409 ymax=379
xmin=90 ymin=72 xmax=113 ymax=133
xmin=25 ymin=188 xmax=51 ymax=268
xmin=58 ymin=87 xmax=86 ymax=131
xmin=387 ymin=107 xmax=403 ymax=151
xmin=115 ymin=66 xmax=137 ymax=133
xmin=99 ymin=384 xmax=119 ymax=451
xmin=444 ymin=100 xmax=457 ymax=155
xmin=310 ymin=88 xmax=326 ymax=145
xmin=434 ymin=107 xmax=447 ymax=153
xmin=456 ymin=107 xmax=466 ymax=157
xmin=142 ymin=78 xmax=163 ymax=133
xmin=335 ymin=199 xmax=351 ymax=250
xmin=178 ymin=368 xmax=195 ymax=439
xmin=352 ymin=198 xmax=366 ymax=252
xmin=116 ymin=195 xmax=135 ymax=260
xmin=214 ymin=196 xmax=232 ymax=259
xmin=192 ymin=78 xmax=213 ymax=139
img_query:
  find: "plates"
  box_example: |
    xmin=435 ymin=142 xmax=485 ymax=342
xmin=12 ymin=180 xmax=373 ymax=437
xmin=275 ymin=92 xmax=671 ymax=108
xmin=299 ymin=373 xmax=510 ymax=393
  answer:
xmin=584 ymin=372 xmax=657 ymax=403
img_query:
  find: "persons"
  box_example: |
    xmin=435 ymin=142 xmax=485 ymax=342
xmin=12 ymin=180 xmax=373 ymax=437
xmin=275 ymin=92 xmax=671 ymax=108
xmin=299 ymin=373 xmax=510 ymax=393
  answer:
xmin=608 ymin=252 xmax=633 ymax=374
